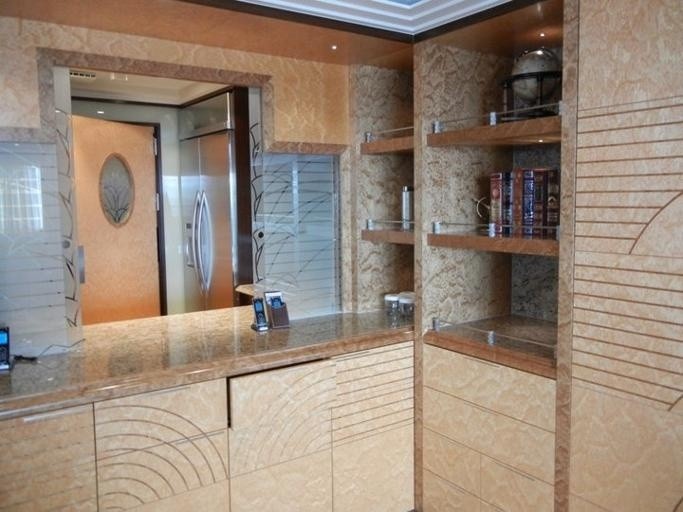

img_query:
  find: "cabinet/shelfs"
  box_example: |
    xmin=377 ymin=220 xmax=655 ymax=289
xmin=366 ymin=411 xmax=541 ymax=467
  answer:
xmin=382 ymin=290 xmax=415 ymax=316
xmin=400 ymin=185 xmax=413 ymax=229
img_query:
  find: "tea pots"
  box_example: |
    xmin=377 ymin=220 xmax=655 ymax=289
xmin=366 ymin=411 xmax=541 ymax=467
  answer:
xmin=50 ymin=64 xmax=250 ymax=329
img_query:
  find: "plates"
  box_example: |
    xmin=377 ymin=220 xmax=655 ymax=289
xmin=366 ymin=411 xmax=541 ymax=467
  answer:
xmin=361 ymin=125 xmax=415 ymax=245
xmin=0 ymin=339 xmax=416 ymax=512
xmin=421 ymin=101 xmax=561 ymax=382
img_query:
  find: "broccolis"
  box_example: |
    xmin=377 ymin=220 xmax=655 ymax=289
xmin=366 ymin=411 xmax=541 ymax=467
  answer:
xmin=174 ymin=90 xmax=255 ymax=314
